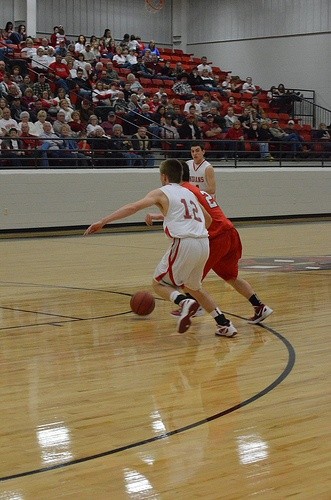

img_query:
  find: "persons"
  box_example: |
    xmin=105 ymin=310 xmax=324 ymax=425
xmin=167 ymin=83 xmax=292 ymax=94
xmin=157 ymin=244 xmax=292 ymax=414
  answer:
xmin=83 ymin=160 xmax=238 ymax=338
xmin=184 ymin=143 xmax=216 ymax=201
xmin=0 ymin=21 xmax=331 ymax=168
xmin=145 ymin=162 xmax=274 ymax=324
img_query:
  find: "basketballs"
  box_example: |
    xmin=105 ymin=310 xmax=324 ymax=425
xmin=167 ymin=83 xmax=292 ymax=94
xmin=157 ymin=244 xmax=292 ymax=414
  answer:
xmin=130 ymin=290 xmax=155 ymax=316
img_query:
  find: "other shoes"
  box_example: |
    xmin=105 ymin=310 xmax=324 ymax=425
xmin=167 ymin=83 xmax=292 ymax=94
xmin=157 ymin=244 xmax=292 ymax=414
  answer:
xmin=302 ymin=149 xmax=312 ymax=158
xmin=228 ymin=158 xmax=239 ymax=162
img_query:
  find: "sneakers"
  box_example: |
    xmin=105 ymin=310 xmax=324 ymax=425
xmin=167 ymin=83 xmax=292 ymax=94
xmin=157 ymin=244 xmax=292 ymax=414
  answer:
xmin=176 ymin=299 xmax=200 ymax=333
xmin=170 ymin=304 xmax=202 ymax=317
xmin=247 ymin=302 xmax=274 ymax=324
xmin=215 ymin=319 xmax=238 ymax=338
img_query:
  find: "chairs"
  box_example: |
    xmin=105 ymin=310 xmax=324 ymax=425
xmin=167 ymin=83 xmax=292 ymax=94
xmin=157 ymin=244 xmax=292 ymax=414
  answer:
xmin=0 ymin=35 xmax=325 ymax=155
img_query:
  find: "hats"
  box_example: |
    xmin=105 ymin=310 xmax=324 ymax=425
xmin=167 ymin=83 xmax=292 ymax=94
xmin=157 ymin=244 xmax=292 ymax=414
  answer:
xmin=108 ymin=111 xmax=115 ymax=116
xmin=320 ymin=123 xmax=329 ymax=131
xmin=38 ymin=72 xmax=46 ymax=78
xmin=166 ymin=113 xmax=176 ymax=119
xmin=271 ymin=118 xmax=279 ymax=124
xmin=185 ymin=113 xmax=195 ymax=119
xmin=13 ymin=96 xmax=21 ymax=100
xmin=206 ymin=114 xmax=213 ymax=118
xmin=288 ymin=120 xmax=295 ymax=125
